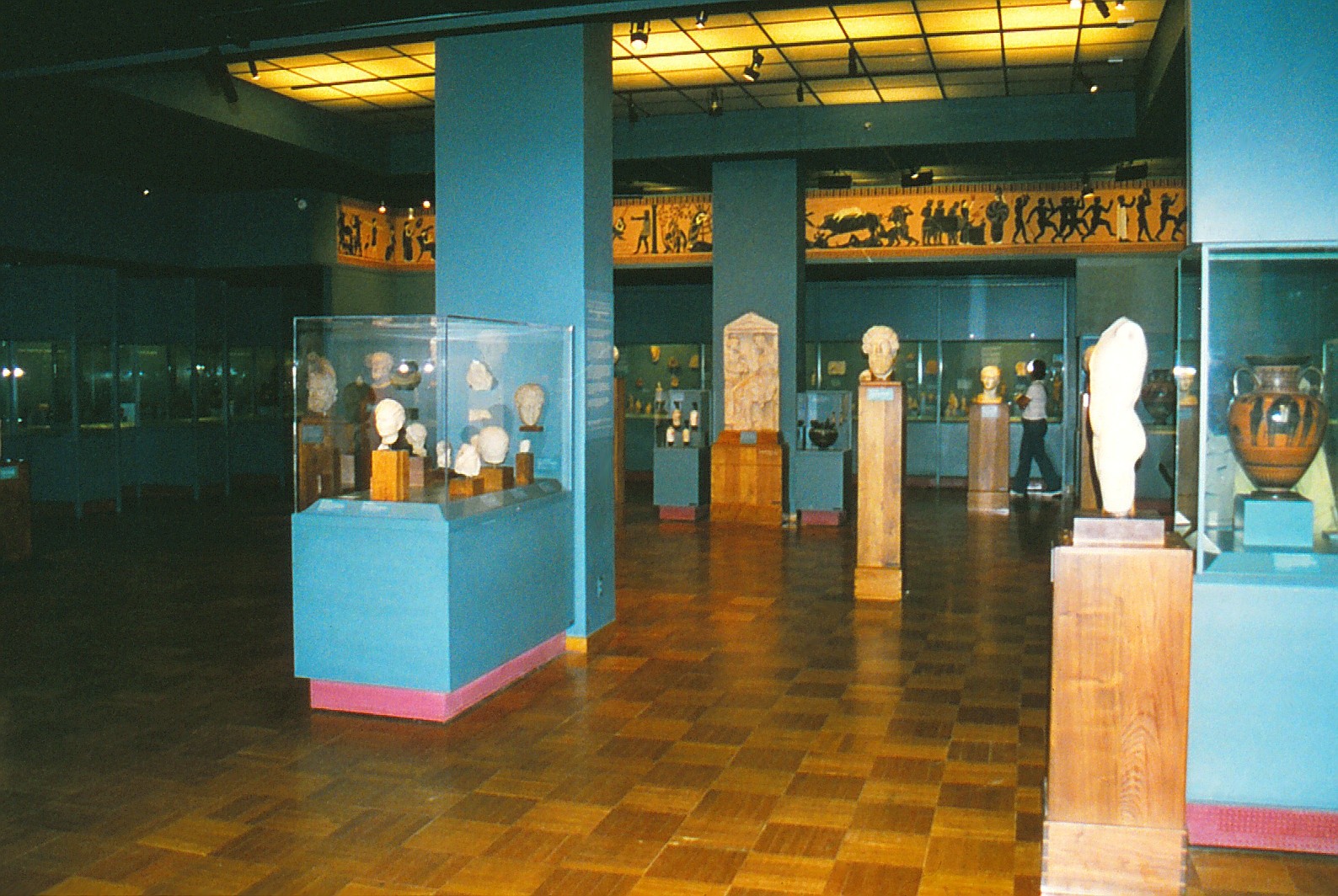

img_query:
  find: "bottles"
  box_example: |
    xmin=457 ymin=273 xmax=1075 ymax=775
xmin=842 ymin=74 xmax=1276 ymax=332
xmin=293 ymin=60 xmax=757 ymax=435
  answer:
xmin=809 ymin=421 xmax=838 ymax=447
xmin=1141 ymin=370 xmax=1176 ymax=425
xmin=1225 ymin=355 xmax=1329 ymax=492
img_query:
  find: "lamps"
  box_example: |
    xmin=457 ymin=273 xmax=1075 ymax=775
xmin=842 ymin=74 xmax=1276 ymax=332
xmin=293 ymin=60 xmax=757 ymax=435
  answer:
xmin=629 ymin=19 xmax=652 ymax=49
xmin=1077 ymin=167 xmax=1095 ymax=198
xmin=818 ymin=174 xmax=852 ymax=189
xmin=1114 ymin=159 xmax=1149 ymax=182
xmin=901 ymin=171 xmax=934 ymax=188
xmin=848 ymin=48 xmax=858 ymax=78
xmin=796 ymin=82 xmax=804 ymax=102
xmin=743 ymin=48 xmax=764 ymax=82
xmin=708 ymin=87 xmax=724 ymax=117
xmin=695 ymin=10 xmax=709 ymax=28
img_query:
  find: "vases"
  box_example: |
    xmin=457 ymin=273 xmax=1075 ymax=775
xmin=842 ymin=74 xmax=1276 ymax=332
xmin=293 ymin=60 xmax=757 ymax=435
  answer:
xmin=1226 ymin=355 xmax=1329 ymax=500
xmin=1141 ymin=369 xmax=1177 ymax=425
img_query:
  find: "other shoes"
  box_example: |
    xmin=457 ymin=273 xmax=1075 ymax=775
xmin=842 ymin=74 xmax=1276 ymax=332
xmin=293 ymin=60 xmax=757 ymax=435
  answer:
xmin=1042 ymin=487 xmax=1062 ymax=497
xmin=1010 ymin=489 xmax=1025 ymax=497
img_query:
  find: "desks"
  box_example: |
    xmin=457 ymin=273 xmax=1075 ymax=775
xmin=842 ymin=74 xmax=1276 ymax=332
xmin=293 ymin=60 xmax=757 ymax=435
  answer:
xmin=792 ymin=448 xmax=850 ymax=526
xmin=1041 ymin=543 xmax=1193 ymax=896
xmin=653 ymin=445 xmax=710 ymax=521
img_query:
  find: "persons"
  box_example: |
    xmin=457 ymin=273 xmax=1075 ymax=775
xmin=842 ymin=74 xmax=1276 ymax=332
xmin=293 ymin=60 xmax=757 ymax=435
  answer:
xmin=1009 ymin=358 xmax=1063 ymax=500
xmin=979 ymin=364 xmax=1000 ymax=399
xmin=862 ymin=326 xmax=902 ymax=382
xmin=614 ymin=343 xmax=702 ymax=446
xmin=801 ymin=348 xmax=1063 ymax=429
xmin=1079 ymin=316 xmax=1197 ymax=520
xmin=304 ymin=348 xmax=546 ymax=478
